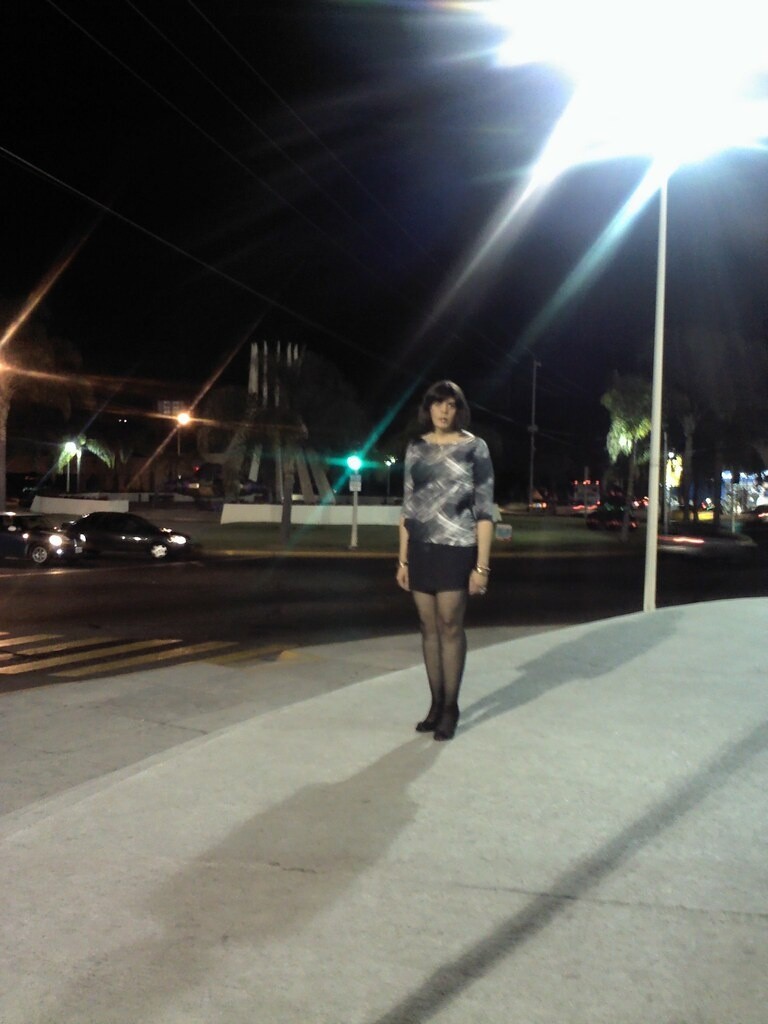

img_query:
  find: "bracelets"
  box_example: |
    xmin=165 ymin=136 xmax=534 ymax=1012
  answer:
xmin=474 ymin=564 xmax=491 ymax=577
xmin=397 ymin=560 xmax=409 ymax=567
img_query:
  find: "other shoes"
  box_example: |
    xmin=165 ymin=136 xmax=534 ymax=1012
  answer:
xmin=416 ymin=702 xmax=443 ymax=732
xmin=433 ymin=703 xmax=460 ymax=741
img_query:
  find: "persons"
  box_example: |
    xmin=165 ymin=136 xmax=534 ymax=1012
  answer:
xmin=396 ymin=379 xmax=495 ymax=741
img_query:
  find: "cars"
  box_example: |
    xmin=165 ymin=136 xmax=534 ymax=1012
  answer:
xmin=59 ymin=511 xmax=192 ymax=561
xmin=738 ymin=504 xmax=768 ymax=525
xmin=0 ymin=510 xmax=89 ymax=564
xmin=671 ymin=504 xmax=715 ymax=521
xmin=587 ymin=510 xmax=639 ymax=530
xmin=526 ymin=500 xmax=547 ymax=512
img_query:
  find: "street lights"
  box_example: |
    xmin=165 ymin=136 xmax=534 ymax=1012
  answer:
xmin=177 ymin=414 xmax=190 ymax=455
xmin=642 ymin=137 xmax=694 ymax=614
xmin=64 ymin=442 xmax=76 ymax=494
xmin=530 ymin=357 xmax=542 ymax=508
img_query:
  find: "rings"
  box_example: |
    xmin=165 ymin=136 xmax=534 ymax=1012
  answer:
xmin=480 ymin=585 xmax=488 ymax=595
